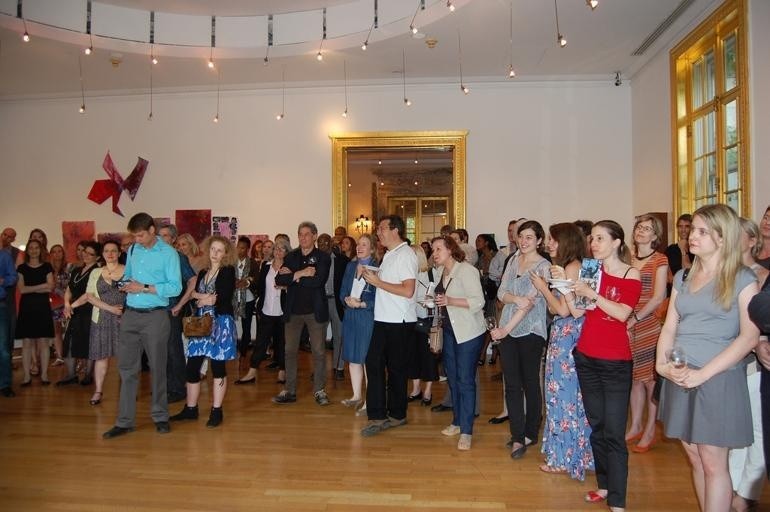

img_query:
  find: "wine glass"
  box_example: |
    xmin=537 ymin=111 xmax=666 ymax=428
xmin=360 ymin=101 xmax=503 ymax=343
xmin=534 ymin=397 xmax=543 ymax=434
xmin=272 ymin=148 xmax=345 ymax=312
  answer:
xmin=665 ymin=347 xmax=696 ymax=392
xmin=306 ymin=256 xmax=319 ymax=280
xmin=531 ymin=269 xmax=547 ymax=298
xmin=482 ymin=317 xmax=501 ymax=345
xmin=433 ymin=292 xmax=447 ymax=319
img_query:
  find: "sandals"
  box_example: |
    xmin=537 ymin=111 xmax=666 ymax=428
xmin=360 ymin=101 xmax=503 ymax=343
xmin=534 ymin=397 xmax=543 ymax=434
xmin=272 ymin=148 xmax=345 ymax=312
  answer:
xmin=540 ymin=463 xmax=571 ymax=474
xmin=585 ymin=489 xmax=607 ymax=504
xmin=624 ymin=430 xmax=656 ymax=453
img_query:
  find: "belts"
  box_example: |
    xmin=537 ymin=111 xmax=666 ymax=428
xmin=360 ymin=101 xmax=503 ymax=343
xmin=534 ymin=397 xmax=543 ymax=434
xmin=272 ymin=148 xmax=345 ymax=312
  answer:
xmin=125 ymin=306 xmax=166 ymax=313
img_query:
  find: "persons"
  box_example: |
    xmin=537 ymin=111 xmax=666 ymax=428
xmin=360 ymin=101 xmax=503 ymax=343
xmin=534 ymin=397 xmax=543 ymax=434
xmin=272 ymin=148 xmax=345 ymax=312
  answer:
xmin=0 ymin=204 xmax=770 ymax=512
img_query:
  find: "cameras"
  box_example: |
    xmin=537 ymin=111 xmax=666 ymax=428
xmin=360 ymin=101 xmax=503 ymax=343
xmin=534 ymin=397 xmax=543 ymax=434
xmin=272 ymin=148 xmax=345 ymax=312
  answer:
xmin=117 ymin=281 xmax=132 ymax=289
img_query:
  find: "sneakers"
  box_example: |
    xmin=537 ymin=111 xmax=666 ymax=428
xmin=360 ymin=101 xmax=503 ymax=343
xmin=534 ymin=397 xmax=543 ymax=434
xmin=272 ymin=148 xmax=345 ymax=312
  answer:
xmin=170 ymin=405 xmax=197 ymax=420
xmin=207 ymin=407 xmax=222 ymax=427
xmin=360 ymin=413 xmax=404 ymax=436
xmin=235 ymin=360 xmax=367 ymax=416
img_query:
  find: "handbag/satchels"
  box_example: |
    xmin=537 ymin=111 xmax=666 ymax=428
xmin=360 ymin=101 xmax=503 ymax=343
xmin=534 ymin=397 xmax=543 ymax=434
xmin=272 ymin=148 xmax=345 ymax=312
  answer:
xmin=181 ymin=315 xmax=211 ymax=336
xmin=427 ymin=324 xmax=444 ymax=354
xmin=654 ymin=297 xmax=669 ymax=322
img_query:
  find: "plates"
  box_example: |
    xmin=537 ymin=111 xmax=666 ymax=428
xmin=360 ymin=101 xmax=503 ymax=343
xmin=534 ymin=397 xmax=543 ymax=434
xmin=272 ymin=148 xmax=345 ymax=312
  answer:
xmin=548 ymin=278 xmax=574 ymax=286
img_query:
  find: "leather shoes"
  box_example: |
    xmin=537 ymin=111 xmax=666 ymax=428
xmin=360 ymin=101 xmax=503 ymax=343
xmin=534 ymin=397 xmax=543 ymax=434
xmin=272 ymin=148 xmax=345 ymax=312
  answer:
xmin=103 ymin=426 xmax=134 ymax=437
xmin=17 ymin=358 xmax=93 ymax=385
xmin=90 ymin=391 xmax=103 ymax=404
xmin=155 ymin=422 xmax=169 ymax=433
xmin=409 ymin=390 xmax=538 ymax=458
xmin=2 ymin=387 xmax=14 ymax=397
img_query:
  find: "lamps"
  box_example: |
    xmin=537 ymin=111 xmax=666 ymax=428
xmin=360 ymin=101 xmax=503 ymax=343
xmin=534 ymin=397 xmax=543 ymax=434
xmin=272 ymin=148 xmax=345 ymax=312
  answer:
xmin=263 ymin=49 xmax=270 ymax=65
xmin=78 ymin=56 xmax=86 ymax=114
xmin=554 ymin=1 xmax=569 ymax=48
xmin=408 ymin=1 xmax=425 ymax=34
xmin=457 ymin=30 xmax=470 ymax=95
xmin=20 ymin=18 xmax=30 ymax=42
xmin=212 ymin=70 xmax=221 ymax=121
xmin=149 ymin=45 xmax=159 ymax=65
xmin=361 ymin=23 xmax=374 ymax=50
xmin=350 ymin=214 xmax=373 ymax=237
xmin=316 ymin=40 xmax=323 ymax=61
xmin=276 ymin=72 xmax=285 ymax=120
xmin=585 ymin=0 xmax=599 ymax=10
xmin=341 ymin=59 xmax=349 ymax=118
xmin=505 ymin=1 xmax=520 ymax=80
xmin=83 ymin=35 xmax=94 ymax=55
xmin=208 ymin=49 xmax=215 ymax=68
xmin=446 ymin=1 xmax=455 ymax=11
xmin=147 ymin=73 xmax=154 ymax=120
xmin=402 ymin=47 xmax=412 ymax=107
